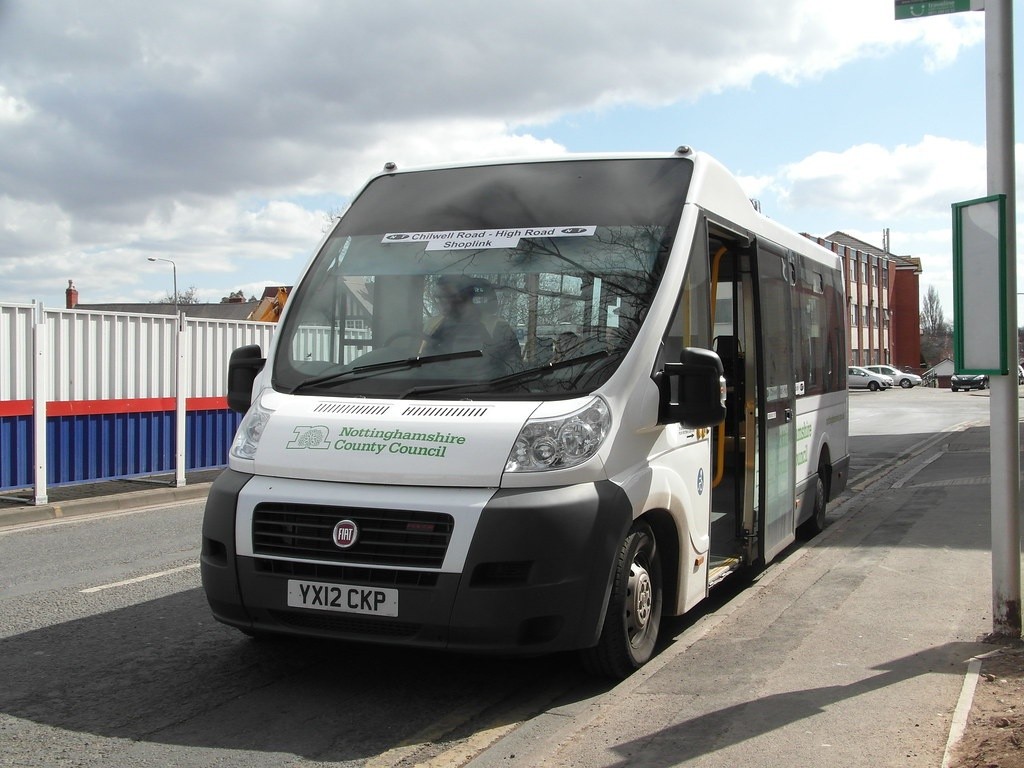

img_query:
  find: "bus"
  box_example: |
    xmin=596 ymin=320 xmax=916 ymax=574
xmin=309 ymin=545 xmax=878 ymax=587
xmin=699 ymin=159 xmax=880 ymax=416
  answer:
xmin=198 ymin=144 xmax=855 ymax=683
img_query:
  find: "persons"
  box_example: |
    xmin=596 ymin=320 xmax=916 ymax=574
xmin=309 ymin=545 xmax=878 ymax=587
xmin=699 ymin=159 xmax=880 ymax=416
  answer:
xmin=522 ymin=332 xmax=582 ymax=379
xmin=409 ymin=277 xmax=507 ymax=379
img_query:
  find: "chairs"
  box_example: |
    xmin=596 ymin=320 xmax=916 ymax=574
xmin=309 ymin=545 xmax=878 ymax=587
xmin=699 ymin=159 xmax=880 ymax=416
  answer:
xmin=473 ymin=278 xmax=521 ymax=376
xmin=522 ymin=332 xmax=579 ymax=369
xmin=711 ymin=336 xmax=743 ymax=393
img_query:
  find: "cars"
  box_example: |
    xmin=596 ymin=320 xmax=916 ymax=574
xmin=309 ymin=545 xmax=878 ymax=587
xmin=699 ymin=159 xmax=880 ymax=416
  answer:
xmin=848 ymin=365 xmax=894 ymax=392
xmin=950 ymin=374 xmax=989 ymax=392
xmin=1018 ymin=364 xmax=1024 ymax=385
xmin=864 ymin=364 xmax=923 ymax=389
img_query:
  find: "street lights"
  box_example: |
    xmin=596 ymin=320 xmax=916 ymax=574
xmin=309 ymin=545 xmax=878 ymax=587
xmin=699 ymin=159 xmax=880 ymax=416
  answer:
xmin=147 ymin=257 xmax=177 ymax=315
